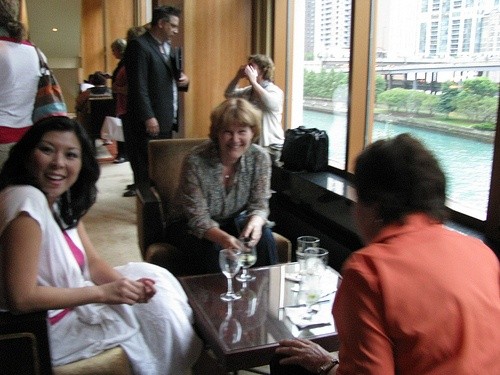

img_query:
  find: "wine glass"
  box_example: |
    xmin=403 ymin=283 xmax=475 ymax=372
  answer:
xmin=219 ymin=300 xmax=242 ymax=344
xmin=219 ymin=249 xmax=243 ymax=302
xmin=297 ymin=236 xmax=329 ymax=319
xmin=236 ymin=281 xmax=257 ymax=317
xmin=235 ymin=237 xmax=257 ymax=282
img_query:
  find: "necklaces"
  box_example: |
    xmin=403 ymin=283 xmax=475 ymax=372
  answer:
xmin=223 ymin=172 xmax=233 ymax=178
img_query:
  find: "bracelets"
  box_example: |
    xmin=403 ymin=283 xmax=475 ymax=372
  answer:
xmin=317 ymin=358 xmax=338 ymax=375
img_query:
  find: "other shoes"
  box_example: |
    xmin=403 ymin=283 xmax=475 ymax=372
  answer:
xmin=123 ymin=189 xmax=136 ymax=197
xmin=126 ymin=185 xmax=134 ymax=189
xmin=114 ymin=157 xmax=130 ymax=163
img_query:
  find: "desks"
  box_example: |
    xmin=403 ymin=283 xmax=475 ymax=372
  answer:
xmin=79 ymin=93 xmax=117 ymax=156
xmin=179 ymin=257 xmax=340 ymax=375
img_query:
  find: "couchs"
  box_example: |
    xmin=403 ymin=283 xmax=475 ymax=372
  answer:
xmin=0 ymin=278 xmax=133 ymax=375
xmin=134 ymin=137 xmax=292 ymax=281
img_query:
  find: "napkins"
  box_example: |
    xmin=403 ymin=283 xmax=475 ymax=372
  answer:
xmin=285 ymin=266 xmax=338 ymax=329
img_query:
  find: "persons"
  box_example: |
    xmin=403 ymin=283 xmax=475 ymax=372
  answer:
xmin=224 ymin=54 xmax=284 ymax=161
xmin=0 ymin=0 xmax=49 ymax=170
xmin=111 ymin=5 xmax=189 ymax=186
xmin=270 ymin=132 xmax=500 ymax=375
xmin=0 ymin=115 xmax=229 ymax=375
xmin=166 ymin=98 xmax=278 ymax=273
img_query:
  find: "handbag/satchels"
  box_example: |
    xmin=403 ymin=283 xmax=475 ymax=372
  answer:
xmin=279 ymin=126 xmax=328 ymax=172
xmin=31 ymin=42 xmax=67 ymax=123
